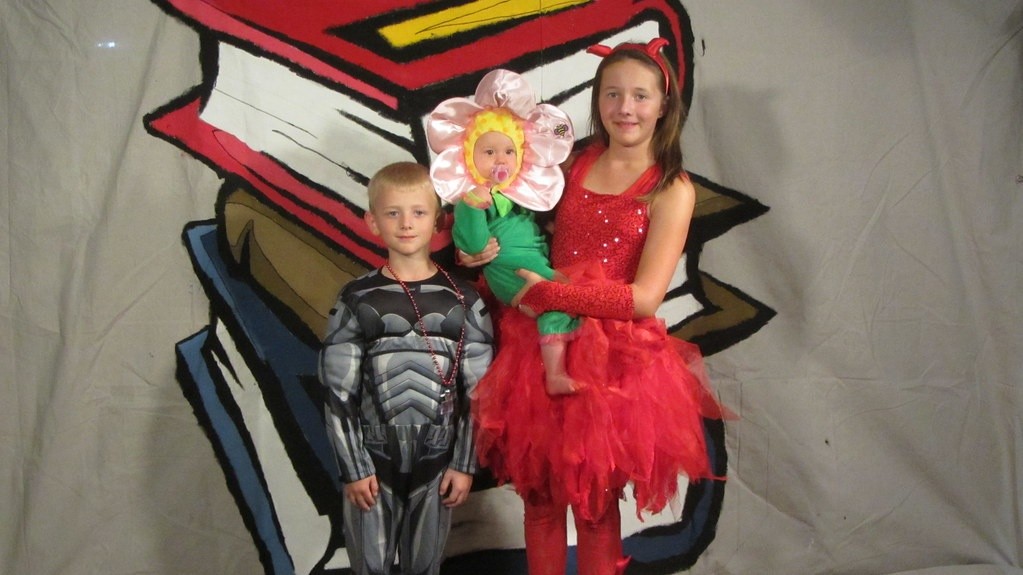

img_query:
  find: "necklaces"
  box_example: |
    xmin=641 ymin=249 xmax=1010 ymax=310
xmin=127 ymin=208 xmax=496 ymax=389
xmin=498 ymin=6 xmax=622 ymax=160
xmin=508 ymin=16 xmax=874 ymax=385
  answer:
xmin=384 ymin=260 xmax=467 ymax=386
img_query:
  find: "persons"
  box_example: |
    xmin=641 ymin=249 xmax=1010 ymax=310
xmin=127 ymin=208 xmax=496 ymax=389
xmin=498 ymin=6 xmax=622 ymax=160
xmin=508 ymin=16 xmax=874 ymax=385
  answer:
xmin=428 ymin=69 xmax=590 ymax=395
xmin=317 ymin=161 xmax=496 ymax=575
xmin=471 ymin=37 xmax=739 ymax=575
